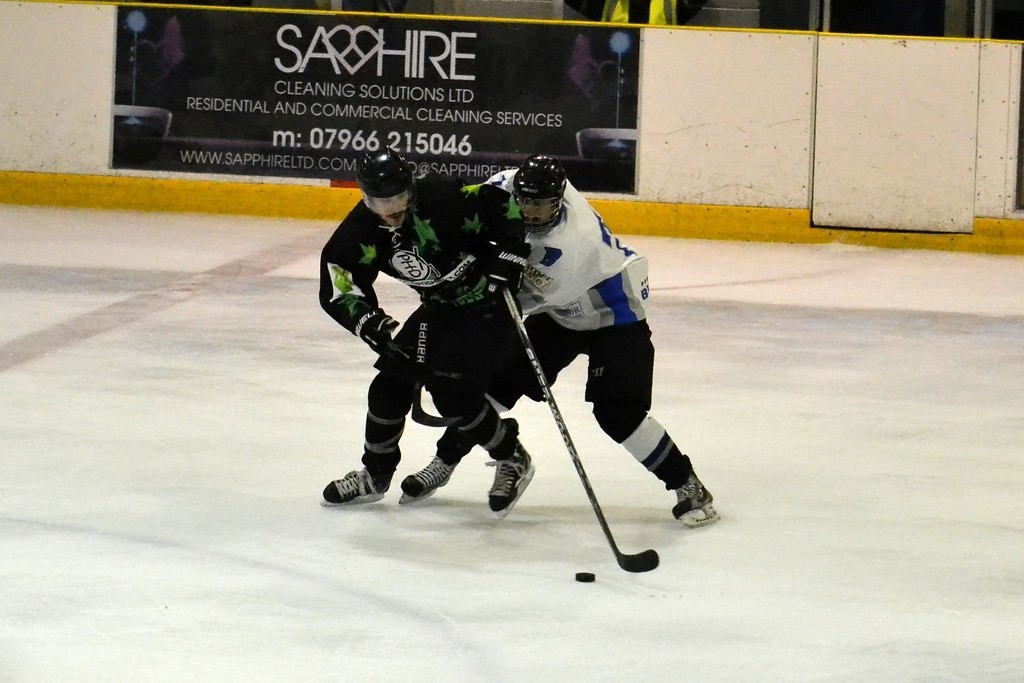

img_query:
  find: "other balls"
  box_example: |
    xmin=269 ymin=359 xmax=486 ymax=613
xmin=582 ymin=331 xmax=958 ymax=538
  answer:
xmin=574 ymin=572 xmax=596 ymax=583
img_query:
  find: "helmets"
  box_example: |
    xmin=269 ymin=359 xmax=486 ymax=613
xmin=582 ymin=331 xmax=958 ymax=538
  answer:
xmin=513 ymin=152 xmax=566 ymax=200
xmin=355 ymin=146 xmax=412 ymax=198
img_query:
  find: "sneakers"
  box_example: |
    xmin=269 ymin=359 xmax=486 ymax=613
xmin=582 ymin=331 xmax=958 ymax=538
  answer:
xmin=484 ymin=418 xmax=535 ymax=520
xmin=321 ymin=465 xmax=396 ymax=507
xmin=672 ymin=454 xmax=720 ymax=528
xmin=399 ymin=455 xmax=461 ymax=504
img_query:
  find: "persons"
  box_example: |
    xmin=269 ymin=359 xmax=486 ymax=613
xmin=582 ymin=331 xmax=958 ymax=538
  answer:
xmin=564 ymin=0 xmax=705 ymax=26
xmin=316 ymin=147 xmax=534 ymax=523
xmin=397 ymin=152 xmax=719 ymax=529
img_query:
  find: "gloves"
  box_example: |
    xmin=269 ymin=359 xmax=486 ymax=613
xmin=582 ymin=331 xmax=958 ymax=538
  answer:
xmin=482 ymin=242 xmax=531 ymax=309
xmin=354 ymin=308 xmax=414 ymax=362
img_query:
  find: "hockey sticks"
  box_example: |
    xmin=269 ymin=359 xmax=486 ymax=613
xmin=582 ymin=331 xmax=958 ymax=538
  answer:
xmin=504 ymin=286 xmax=659 ymax=573
